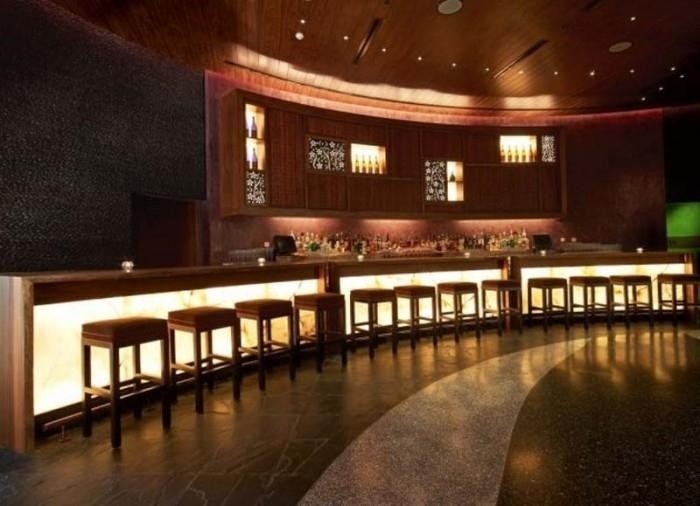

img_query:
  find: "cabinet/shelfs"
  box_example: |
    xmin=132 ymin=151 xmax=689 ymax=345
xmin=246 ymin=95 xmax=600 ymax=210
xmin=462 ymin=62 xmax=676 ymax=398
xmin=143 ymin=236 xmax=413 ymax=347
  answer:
xmin=496 ymin=133 xmax=540 ymax=167
xmin=444 ymin=160 xmax=467 ymax=205
xmin=242 ymin=99 xmax=272 ymax=209
xmin=346 ymin=140 xmax=392 ymax=176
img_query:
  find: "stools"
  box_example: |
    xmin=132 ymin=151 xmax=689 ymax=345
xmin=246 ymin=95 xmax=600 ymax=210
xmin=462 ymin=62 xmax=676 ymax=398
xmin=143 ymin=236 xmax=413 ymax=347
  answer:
xmin=234 ymin=298 xmax=296 ymax=391
xmin=166 ymin=306 xmax=244 ymax=416
xmin=609 ymin=274 xmax=655 ymax=331
xmin=292 ymin=293 xmax=348 ymax=372
xmin=350 ymin=288 xmax=400 ymax=361
xmin=79 ymin=316 xmax=174 ymax=451
xmin=527 ymin=276 xmax=570 ymax=333
xmin=394 ymin=285 xmax=439 ymax=350
xmin=481 ymin=279 xmax=524 ymax=337
xmin=437 ymin=281 xmax=481 ymax=343
xmin=568 ymin=276 xmax=613 ymax=332
xmin=656 ymin=273 xmax=700 ymax=328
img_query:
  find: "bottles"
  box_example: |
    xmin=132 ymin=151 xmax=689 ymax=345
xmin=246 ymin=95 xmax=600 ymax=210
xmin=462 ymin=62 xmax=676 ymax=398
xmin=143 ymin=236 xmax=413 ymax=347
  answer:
xmin=450 ymin=171 xmax=456 ymax=181
xmin=246 ymin=116 xmax=258 ymax=169
xmin=559 ymin=237 xmax=566 ymax=253
xmin=355 ymin=153 xmax=379 ymax=174
xmin=500 ymin=144 xmax=533 ymax=163
xmin=571 ymin=236 xmax=577 ymax=252
xmin=290 ymin=224 xmax=529 ymax=255
xmin=264 ymin=241 xmax=270 ymax=261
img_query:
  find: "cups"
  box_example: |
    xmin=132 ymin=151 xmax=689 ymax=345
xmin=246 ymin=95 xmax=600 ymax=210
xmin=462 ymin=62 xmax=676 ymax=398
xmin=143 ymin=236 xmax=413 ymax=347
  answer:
xmin=636 ymin=248 xmax=643 ymax=253
xmin=121 ymin=261 xmax=134 ymax=272
xmin=540 ymin=250 xmax=546 ymax=256
xmin=227 ymin=248 xmax=265 ymax=262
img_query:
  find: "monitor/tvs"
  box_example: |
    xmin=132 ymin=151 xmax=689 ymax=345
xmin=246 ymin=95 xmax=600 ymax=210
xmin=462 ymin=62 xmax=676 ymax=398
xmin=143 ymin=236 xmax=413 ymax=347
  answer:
xmin=274 ymin=236 xmax=297 ymax=255
xmin=534 ymin=235 xmax=551 ymax=249
